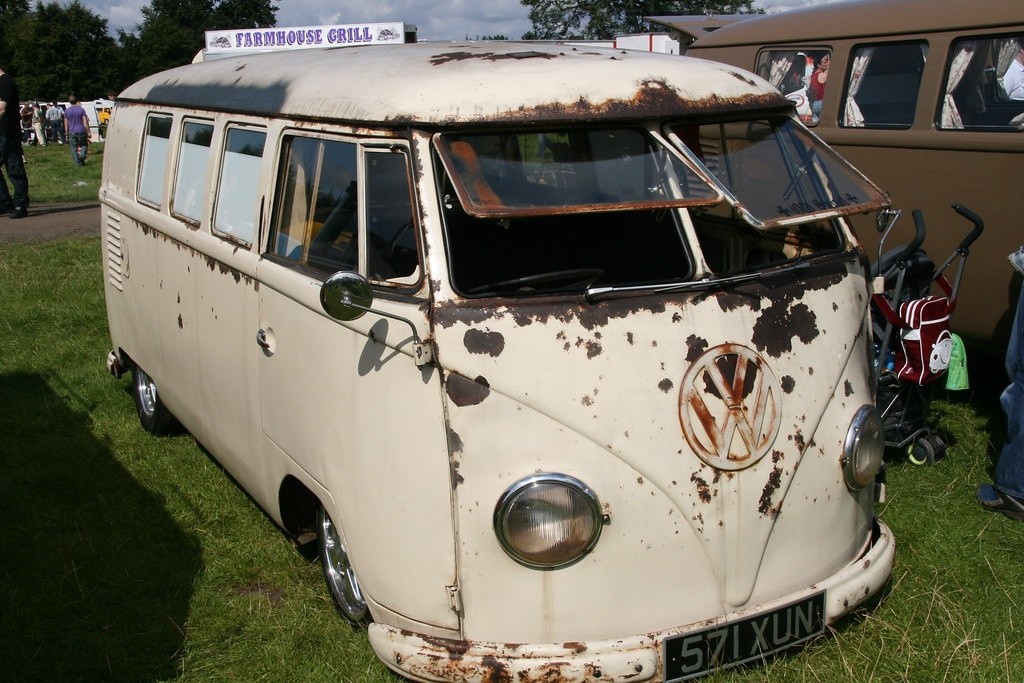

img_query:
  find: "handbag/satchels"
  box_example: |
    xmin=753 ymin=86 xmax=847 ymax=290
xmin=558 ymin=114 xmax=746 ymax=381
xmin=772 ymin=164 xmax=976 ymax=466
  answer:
xmin=874 ymin=269 xmax=952 ymax=386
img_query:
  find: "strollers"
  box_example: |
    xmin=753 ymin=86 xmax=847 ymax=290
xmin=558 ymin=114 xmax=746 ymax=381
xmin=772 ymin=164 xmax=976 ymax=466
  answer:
xmin=866 ymin=203 xmax=986 ymax=466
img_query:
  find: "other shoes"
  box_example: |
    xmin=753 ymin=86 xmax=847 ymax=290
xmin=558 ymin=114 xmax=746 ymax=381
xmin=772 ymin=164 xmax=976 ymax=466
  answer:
xmin=975 ymin=484 xmax=1024 ymax=522
xmin=9 ymin=206 xmax=28 ymax=219
xmin=0 ymin=201 xmax=13 ymax=216
xmin=79 ymin=157 xmax=85 ymax=166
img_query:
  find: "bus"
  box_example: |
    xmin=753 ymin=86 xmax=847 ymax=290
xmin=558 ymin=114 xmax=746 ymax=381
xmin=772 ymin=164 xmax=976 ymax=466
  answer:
xmin=98 ymin=19 xmax=901 ymax=683
xmin=641 ymin=0 xmax=1024 ymax=376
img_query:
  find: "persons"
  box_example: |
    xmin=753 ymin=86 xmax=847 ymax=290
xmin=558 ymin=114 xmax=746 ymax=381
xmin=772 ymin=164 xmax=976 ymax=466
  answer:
xmin=810 ymin=51 xmax=830 ymax=122
xmin=20 ymin=95 xmax=92 ymax=167
xmin=1003 ymin=47 xmax=1024 ymax=100
xmin=0 ymin=65 xmax=29 ymax=219
xmin=531 ymin=134 xmax=555 ymax=186
xmin=759 ymin=63 xmax=768 ymax=81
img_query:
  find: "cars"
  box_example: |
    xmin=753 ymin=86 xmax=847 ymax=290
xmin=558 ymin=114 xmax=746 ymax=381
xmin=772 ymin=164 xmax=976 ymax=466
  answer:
xmin=17 ymin=99 xmax=115 ymax=139
